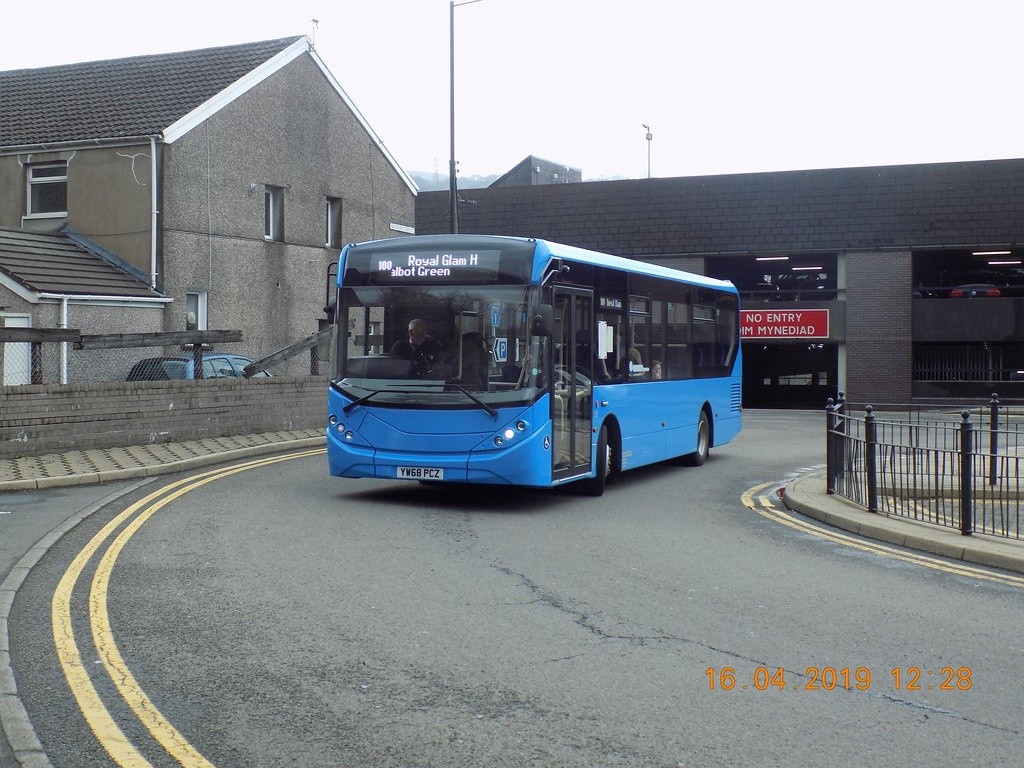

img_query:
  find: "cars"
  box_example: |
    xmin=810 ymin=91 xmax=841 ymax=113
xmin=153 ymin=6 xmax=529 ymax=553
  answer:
xmin=126 ymin=352 xmax=273 ymax=381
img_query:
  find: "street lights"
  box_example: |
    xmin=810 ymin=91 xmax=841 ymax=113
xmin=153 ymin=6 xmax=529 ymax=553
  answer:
xmin=641 ymin=123 xmax=652 ymax=179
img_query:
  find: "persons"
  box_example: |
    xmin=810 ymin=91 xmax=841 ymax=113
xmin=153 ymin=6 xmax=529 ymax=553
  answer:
xmin=652 ymin=359 xmax=664 ymax=380
xmin=389 ymin=318 xmax=442 ymax=380
xmin=628 ymin=347 xmax=645 ymax=383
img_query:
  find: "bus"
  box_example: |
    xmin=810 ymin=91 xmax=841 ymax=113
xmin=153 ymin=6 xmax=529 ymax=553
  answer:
xmin=323 ymin=231 xmax=745 ymax=497
